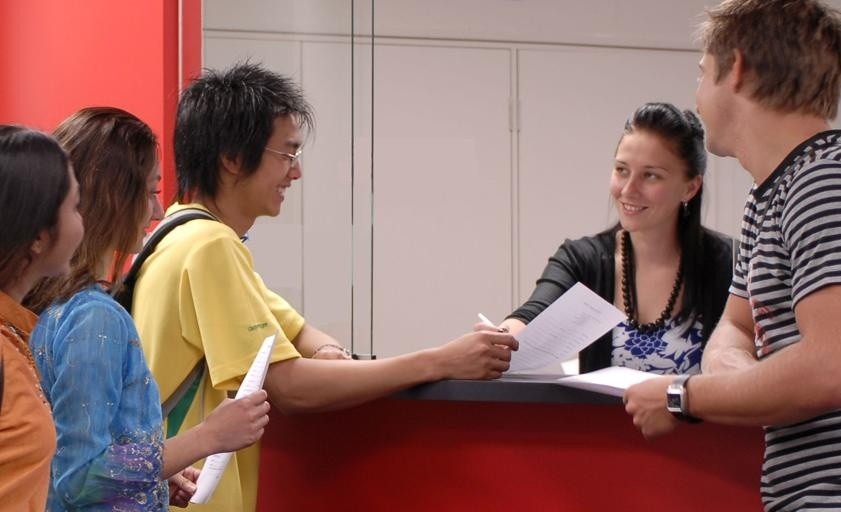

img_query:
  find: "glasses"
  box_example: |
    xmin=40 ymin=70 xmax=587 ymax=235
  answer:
xmin=262 ymin=148 xmax=302 ymax=167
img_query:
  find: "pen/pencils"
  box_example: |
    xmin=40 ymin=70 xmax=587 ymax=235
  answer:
xmin=478 ymin=313 xmax=493 ymax=326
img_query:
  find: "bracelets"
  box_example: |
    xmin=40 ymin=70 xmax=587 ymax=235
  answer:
xmin=309 ymin=344 xmax=349 ymax=355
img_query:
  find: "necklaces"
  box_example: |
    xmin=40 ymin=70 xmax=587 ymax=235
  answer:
xmin=620 ymin=227 xmax=686 ymax=335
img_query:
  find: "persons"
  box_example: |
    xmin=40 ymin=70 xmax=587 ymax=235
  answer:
xmin=31 ymin=106 xmax=274 ymax=511
xmin=128 ymin=56 xmax=518 ymax=512
xmin=472 ymin=100 xmax=742 ymax=375
xmin=620 ymin=2 xmax=840 ymax=512
xmin=1 ymin=123 xmax=86 ymax=512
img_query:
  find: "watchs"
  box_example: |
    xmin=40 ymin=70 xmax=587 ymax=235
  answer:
xmin=667 ymin=370 xmax=704 ymax=424
